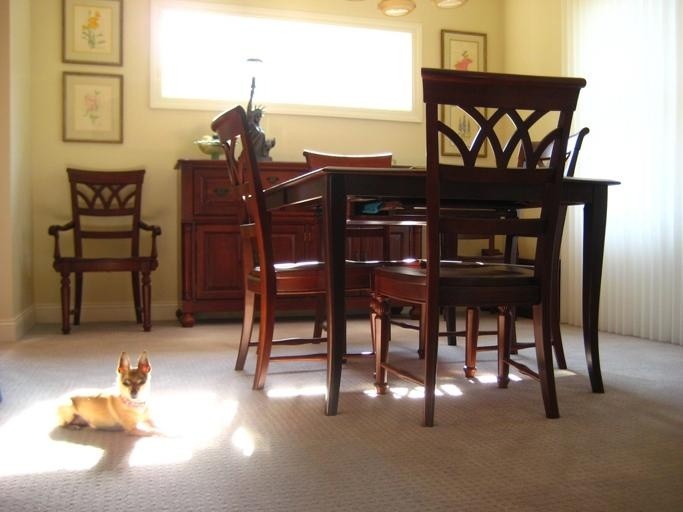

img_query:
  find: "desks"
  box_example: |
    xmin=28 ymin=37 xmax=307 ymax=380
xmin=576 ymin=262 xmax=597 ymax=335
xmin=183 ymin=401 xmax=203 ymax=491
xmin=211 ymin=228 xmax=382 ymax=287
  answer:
xmin=262 ymin=166 xmax=620 ymax=416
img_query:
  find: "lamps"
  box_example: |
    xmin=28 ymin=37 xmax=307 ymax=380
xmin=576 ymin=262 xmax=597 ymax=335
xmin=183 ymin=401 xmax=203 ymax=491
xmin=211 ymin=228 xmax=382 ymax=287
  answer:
xmin=380 ymin=0 xmax=415 ymax=17
xmin=435 ymin=0 xmax=466 ymax=10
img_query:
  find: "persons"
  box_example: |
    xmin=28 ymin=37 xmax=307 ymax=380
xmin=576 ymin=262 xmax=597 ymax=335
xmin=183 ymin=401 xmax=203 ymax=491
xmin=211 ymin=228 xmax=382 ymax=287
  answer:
xmin=245 ymin=76 xmax=276 ymax=162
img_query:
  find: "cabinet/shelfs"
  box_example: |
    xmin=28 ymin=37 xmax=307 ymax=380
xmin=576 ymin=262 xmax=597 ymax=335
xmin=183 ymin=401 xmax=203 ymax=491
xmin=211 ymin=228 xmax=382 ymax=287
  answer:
xmin=174 ymin=159 xmax=423 ymax=327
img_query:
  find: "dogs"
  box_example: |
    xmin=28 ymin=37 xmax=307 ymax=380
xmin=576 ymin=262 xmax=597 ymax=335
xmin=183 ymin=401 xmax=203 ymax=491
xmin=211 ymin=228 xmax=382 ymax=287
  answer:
xmin=58 ymin=350 xmax=163 ymax=437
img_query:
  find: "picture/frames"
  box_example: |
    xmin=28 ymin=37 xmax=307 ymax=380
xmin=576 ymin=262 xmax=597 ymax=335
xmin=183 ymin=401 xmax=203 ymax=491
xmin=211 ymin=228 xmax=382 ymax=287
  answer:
xmin=63 ymin=71 xmax=123 ymax=143
xmin=62 ymin=0 xmax=123 ymax=65
xmin=441 ymin=102 xmax=488 ymax=157
xmin=441 ymin=29 xmax=488 ymax=72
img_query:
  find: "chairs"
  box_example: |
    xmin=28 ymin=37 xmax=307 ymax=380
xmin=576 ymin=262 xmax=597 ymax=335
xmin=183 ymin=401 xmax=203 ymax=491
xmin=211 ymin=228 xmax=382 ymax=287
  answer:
xmin=49 ymin=168 xmax=161 ymax=334
xmin=209 ymin=104 xmax=395 ymax=396
xmin=465 ymin=129 xmax=587 ymax=385
xmin=368 ymin=68 xmax=588 ymax=425
xmin=301 ymin=148 xmax=409 ymax=347
